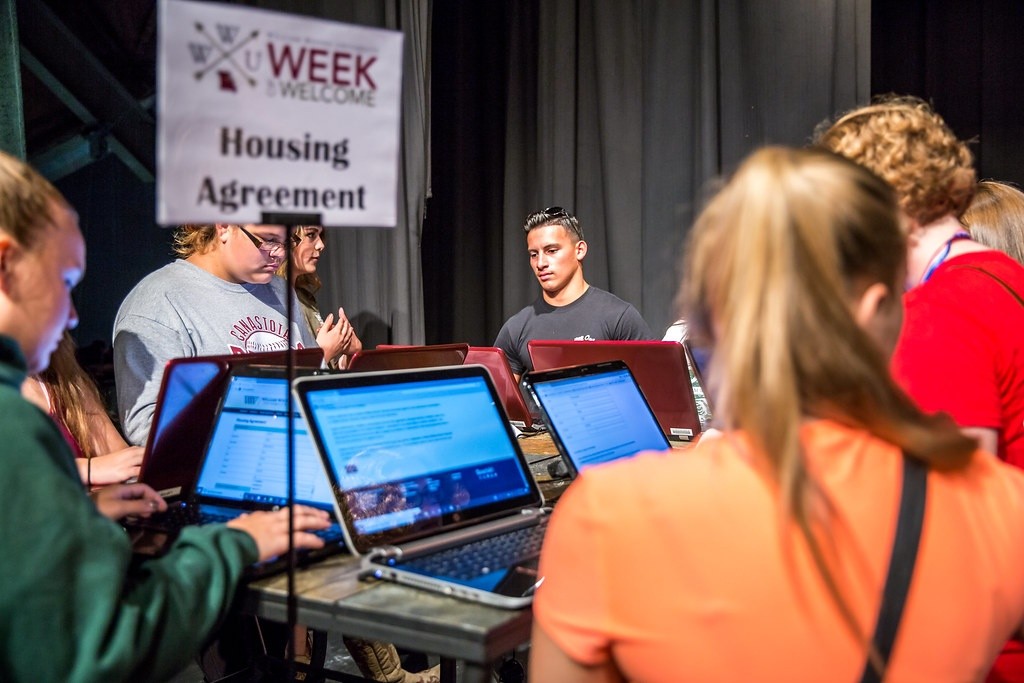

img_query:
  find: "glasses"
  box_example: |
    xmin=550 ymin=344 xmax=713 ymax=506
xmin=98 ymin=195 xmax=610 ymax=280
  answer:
xmin=236 ymin=225 xmax=301 ymax=251
xmin=525 ymin=205 xmax=582 ymax=243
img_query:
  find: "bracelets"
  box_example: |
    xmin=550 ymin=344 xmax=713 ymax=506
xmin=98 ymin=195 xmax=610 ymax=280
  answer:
xmin=88 ymin=456 xmax=92 ymax=486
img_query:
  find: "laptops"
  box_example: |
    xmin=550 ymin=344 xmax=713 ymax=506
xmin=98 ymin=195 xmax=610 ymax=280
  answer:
xmin=126 ymin=339 xmax=721 ymax=608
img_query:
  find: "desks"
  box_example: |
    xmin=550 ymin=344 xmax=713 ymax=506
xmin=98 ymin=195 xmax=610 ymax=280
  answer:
xmin=249 ymin=433 xmax=692 ymax=683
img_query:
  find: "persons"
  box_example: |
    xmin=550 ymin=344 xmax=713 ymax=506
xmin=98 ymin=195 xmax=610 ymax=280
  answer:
xmin=0 ymin=150 xmax=335 ymax=682
xmin=20 ymin=331 xmax=149 ymax=486
xmin=113 ymin=223 xmax=442 ymax=683
xmin=494 ymin=208 xmax=657 ymax=381
xmin=526 ymin=146 xmax=1024 ymax=683
xmin=665 ymin=97 xmax=1024 ymax=683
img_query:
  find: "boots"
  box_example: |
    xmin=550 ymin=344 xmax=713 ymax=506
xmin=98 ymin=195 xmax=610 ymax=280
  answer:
xmin=343 ymin=638 xmax=442 ymax=682
